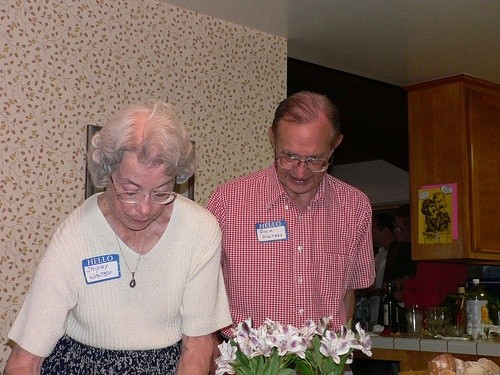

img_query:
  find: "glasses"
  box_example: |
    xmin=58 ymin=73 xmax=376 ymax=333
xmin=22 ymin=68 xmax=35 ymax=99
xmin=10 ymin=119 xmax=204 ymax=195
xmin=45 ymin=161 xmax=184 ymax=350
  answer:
xmin=274 ymin=149 xmax=334 ymax=173
xmin=110 ymin=173 xmax=178 ymax=205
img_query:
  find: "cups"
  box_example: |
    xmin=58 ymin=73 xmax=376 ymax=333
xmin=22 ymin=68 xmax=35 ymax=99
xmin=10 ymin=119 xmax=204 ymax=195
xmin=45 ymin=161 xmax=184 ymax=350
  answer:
xmin=407 ymin=312 xmax=423 ymax=337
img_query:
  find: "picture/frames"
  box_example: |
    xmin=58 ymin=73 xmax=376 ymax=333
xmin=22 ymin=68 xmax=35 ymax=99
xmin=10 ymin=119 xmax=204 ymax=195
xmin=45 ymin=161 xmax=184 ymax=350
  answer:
xmin=84 ymin=124 xmax=195 ymax=203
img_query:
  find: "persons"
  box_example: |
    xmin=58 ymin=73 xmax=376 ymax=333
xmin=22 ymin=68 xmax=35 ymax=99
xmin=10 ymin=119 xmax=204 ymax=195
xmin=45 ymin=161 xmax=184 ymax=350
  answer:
xmin=363 ymin=204 xmax=467 ymax=332
xmin=4 ymin=101 xmax=233 ymax=375
xmin=205 ymin=92 xmax=376 ymax=375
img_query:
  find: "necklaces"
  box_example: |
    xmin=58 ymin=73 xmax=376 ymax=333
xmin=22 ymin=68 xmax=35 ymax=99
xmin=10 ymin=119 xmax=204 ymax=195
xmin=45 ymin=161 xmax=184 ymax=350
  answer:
xmin=107 ymin=202 xmax=150 ymax=288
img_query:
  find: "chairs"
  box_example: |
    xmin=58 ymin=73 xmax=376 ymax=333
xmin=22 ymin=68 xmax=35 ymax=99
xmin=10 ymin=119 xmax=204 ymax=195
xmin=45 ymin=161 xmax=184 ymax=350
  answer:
xmin=349 ymin=357 xmax=400 ymax=375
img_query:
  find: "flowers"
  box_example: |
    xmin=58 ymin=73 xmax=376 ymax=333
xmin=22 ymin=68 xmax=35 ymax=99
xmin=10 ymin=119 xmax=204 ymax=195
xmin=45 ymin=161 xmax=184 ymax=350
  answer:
xmin=215 ymin=316 xmax=372 ymax=375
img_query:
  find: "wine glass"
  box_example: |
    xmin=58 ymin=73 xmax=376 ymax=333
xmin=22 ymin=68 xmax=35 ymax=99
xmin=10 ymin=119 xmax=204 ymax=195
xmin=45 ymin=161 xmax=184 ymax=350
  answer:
xmin=426 ymin=307 xmax=453 ymax=335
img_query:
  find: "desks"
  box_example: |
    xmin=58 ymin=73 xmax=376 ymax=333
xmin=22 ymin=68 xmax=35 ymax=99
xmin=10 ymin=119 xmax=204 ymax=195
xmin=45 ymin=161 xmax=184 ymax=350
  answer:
xmin=371 ymin=335 xmax=500 ymax=375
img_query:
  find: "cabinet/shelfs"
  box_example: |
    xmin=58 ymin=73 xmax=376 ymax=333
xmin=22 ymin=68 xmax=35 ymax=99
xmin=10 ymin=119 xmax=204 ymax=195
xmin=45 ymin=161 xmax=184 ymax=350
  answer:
xmin=407 ymin=74 xmax=500 ymax=265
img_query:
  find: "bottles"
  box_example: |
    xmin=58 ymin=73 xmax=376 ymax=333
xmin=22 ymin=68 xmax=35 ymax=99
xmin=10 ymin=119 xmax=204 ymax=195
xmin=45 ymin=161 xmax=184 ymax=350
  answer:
xmin=466 ymin=278 xmax=489 ymax=341
xmin=454 ymin=286 xmax=467 ymax=335
xmin=382 ymin=283 xmax=397 ymax=332
